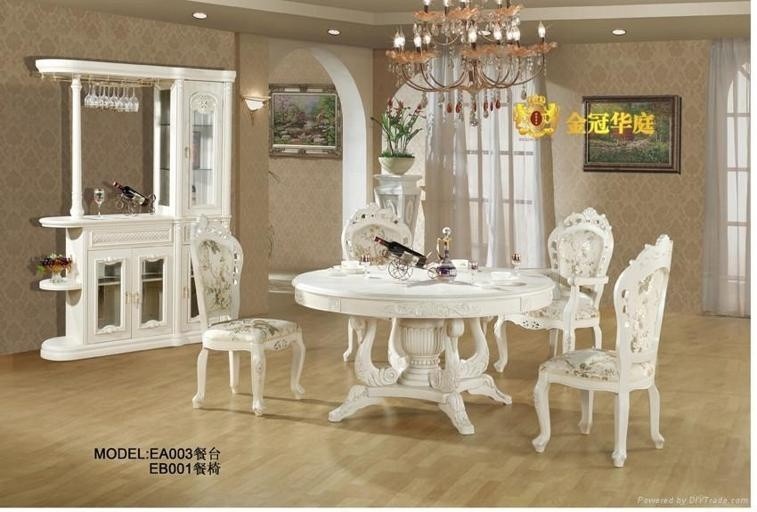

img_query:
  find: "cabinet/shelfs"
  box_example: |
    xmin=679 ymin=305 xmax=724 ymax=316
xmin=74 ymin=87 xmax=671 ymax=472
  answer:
xmin=152 ymin=81 xmax=233 ymax=218
xmin=183 ymin=221 xmax=221 ymax=339
xmin=38 ymin=214 xmax=172 ymax=361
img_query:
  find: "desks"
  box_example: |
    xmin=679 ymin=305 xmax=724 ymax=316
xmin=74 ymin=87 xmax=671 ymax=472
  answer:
xmin=292 ymin=263 xmax=555 ymax=434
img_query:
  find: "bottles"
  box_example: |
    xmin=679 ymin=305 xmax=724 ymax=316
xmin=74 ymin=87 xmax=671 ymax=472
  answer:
xmin=374 ymin=236 xmax=426 ymax=268
xmin=113 ymin=182 xmax=149 ymax=207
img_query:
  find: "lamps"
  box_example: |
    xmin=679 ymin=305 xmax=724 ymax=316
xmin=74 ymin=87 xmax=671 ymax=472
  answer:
xmin=384 ymin=0 xmax=558 ymax=127
xmin=242 ymin=94 xmax=268 ymax=124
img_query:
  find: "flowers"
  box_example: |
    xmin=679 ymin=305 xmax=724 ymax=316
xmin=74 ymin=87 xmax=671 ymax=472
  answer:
xmin=370 ymin=98 xmax=426 ymax=155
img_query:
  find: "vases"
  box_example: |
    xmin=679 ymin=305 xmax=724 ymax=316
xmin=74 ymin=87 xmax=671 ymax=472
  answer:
xmin=379 ymin=155 xmax=414 ymax=174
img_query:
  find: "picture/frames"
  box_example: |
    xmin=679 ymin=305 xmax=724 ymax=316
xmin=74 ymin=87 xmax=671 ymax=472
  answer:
xmin=582 ymin=95 xmax=681 ymax=175
xmin=267 ymin=83 xmax=342 ymax=159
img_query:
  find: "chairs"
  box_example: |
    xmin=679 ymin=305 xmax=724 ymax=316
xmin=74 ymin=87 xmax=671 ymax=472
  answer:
xmin=532 ymin=233 xmax=674 ymax=469
xmin=493 ymin=205 xmax=609 ymax=372
xmin=339 ymin=203 xmax=414 ymax=363
xmin=191 ymin=214 xmax=306 ymax=417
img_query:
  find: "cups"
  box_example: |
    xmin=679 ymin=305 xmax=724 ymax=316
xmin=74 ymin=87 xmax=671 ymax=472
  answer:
xmin=342 ymin=261 xmax=359 ymax=274
xmin=491 ymin=272 xmax=511 ymax=286
xmin=451 ymin=260 xmax=468 ymax=272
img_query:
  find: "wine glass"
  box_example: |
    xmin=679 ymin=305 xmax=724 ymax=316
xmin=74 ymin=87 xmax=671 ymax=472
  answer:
xmin=512 ymin=252 xmax=521 ymax=276
xmin=93 ymin=188 xmax=105 ymax=220
xmin=470 ymin=262 xmax=479 ymax=281
xmin=362 ymin=254 xmax=371 ymax=280
xmin=84 ymin=80 xmax=139 ymax=112
